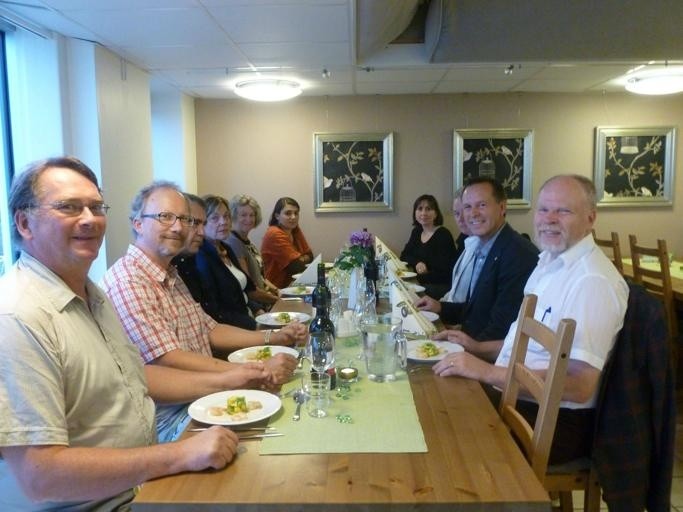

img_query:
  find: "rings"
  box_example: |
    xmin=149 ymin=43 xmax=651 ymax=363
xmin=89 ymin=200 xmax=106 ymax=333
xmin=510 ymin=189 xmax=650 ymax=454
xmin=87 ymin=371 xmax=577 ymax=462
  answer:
xmin=449 ymin=359 xmax=454 ymax=367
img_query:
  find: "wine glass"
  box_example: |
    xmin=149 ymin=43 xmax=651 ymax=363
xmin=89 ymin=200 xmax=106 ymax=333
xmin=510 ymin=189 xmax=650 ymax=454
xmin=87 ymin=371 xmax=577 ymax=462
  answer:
xmin=302 ymin=258 xmax=379 ymax=419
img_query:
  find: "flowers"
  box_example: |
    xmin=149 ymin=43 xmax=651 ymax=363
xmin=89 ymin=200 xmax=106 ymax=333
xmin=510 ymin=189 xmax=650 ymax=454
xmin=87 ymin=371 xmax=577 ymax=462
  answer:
xmin=331 ymin=229 xmax=375 ymax=270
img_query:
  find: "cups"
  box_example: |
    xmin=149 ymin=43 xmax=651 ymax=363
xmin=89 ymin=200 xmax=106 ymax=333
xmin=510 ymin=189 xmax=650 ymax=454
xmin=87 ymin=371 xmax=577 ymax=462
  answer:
xmin=304 ymin=371 xmax=331 ymax=418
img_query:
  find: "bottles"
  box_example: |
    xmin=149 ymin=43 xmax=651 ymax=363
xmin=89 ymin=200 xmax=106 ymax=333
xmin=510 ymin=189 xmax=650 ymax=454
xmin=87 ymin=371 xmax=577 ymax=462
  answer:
xmin=312 ymin=263 xmax=331 ymax=319
xmin=309 ymin=297 xmax=336 ymax=389
xmin=364 ymin=254 xmax=380 ymax=304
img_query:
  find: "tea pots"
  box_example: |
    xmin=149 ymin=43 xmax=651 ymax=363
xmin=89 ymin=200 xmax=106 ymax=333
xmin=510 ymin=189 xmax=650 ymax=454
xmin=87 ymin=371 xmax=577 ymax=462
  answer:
xmin=359 ymin=315 xmax=408 ymax=383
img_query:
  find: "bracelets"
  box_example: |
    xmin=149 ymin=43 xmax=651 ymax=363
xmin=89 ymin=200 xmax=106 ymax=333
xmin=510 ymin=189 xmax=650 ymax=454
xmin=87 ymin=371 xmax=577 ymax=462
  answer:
xmin=262 ymin=327 xmax=275 ymax=344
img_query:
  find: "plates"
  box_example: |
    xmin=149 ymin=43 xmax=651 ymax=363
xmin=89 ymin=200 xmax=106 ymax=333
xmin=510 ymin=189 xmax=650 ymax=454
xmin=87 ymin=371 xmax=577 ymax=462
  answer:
xmin=227 ymin=345 xmax=300 ymax=367
xmin=281 ymin=261 xmax=332 ymax=296
xmin=397 ymin=271 xmax=418 ymax=278
xmin=255 ymin=312 xmax=310 ymax=325
xmin=187 ymin=389 xmax=283 ymax=426
xmin=384 ymin=310 xmax=439 ymax=324
xmin=398 ymin=339 xmax=466 ymax=362
xmin=402 ymin=284 xmax=426 ymax=292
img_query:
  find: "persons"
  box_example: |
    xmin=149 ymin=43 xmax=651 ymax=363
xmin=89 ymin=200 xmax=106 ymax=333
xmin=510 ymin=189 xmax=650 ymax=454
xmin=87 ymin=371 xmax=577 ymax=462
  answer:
xmin=436 ymin=184 xmax=480 ymax=331
xmin=430 ymin=173 xmax=630 ymax=466
xmin=173 ymin=190 xmax=314 ymax=330
xmin=415 ymin=178 xmax=539 ymax=402
xmin=99 ymin=180 xmax=308 ymax=445
xmin=400 ymin=194 xmax=455 ymax=299
xmin=0 ymin=155 xmax=237 ymax=512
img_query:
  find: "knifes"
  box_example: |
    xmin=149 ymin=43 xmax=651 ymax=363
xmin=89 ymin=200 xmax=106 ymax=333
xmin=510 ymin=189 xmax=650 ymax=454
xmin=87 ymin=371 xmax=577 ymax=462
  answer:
xmin=235 ymin=433 xmax=291 ymax=441
xmin=186 ymin=425 xmax=277 ymax=431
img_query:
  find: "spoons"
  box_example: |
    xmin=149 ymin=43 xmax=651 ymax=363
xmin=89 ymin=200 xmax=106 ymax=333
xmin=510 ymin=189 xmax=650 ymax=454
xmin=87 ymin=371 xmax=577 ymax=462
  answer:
xmin=293 ymin=389 xmax=305 ymax=420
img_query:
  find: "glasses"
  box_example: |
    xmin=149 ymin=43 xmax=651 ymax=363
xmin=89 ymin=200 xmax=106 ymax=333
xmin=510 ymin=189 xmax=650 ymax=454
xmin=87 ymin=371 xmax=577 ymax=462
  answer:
xmin=140 ymin=212 xmax=193 ymax=227
xmin=38 ymin=201 xmax=110 ymax=215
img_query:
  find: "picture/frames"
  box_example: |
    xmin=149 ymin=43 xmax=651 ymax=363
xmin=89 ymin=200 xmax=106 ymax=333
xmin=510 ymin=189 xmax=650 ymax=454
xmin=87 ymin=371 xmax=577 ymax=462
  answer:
xmin=313 ymin=130 xmax=396 ymax=214
xmin=450 ymin=127 xmax=534 ymax=212
xmin=593 ymin=124 xmax=678 ymax=210
